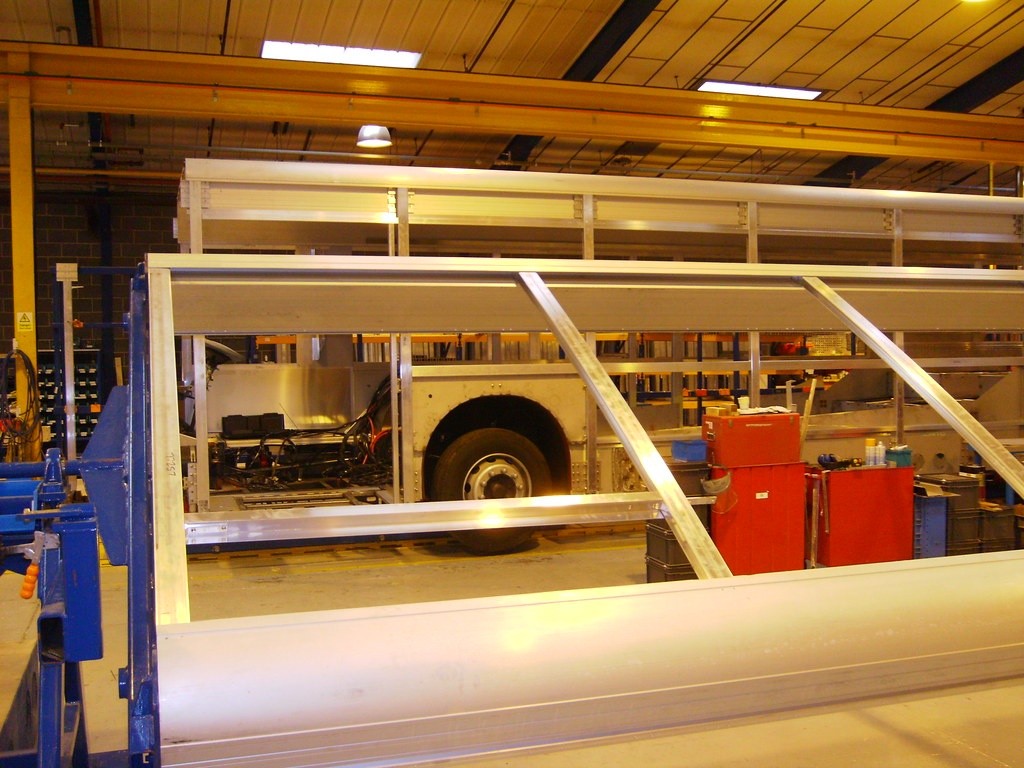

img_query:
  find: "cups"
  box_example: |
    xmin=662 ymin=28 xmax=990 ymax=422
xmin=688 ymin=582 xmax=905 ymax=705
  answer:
xmin=737 ymin=397 xmax=750 ymax=411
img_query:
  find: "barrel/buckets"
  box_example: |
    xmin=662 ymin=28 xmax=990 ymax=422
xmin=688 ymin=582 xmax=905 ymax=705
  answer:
xmin=959 ymin=466 xmax=985 ymax=502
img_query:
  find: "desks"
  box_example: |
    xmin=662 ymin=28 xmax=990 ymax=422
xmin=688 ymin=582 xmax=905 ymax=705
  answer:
xmin=208 ymin=429 xmax=360 ymax=448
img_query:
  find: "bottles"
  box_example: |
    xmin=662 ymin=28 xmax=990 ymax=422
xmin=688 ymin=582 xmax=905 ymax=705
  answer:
xmin=876 ymin=442 xmax=886 ymax=466
xmin=865 ymin=439 xmax=876 ymax=466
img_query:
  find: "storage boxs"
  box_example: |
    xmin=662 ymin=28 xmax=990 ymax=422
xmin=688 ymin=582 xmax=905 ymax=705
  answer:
xmin=980 ymin=501 xmax=1016 ymax=540
xmin=706 ymin=407 xmax=727 ymax=417
xmin=645 ymin=557 xmax=698 ymax=584
xmin=644 ymin=505 xmax=708 ymax=532
xmin=722 ymin=403 xmax=738 ymax=414
xmin=645 ymin=525 xmax=710 ymax=566
xmin=946 ymin=509 xmax=980 ymax=543
xmin=983 ymin=539 xmax=1016 ymax=553
xmin=946 ymin=541 xmax=982 ymax=557
xmin=887 ymin=449 xmax=913 ymax=466
xmin=673 ymin=439 xmax=707 ymax=461
xmin=917 ymin=473 xmax=983 ymax=511
xmin=666 ymin=460 xmax=712 ymax=495
xmin=701 ymin=414 xmax=803 ymax=464
xmin=915 ymin=497 xmax=948 ymax=561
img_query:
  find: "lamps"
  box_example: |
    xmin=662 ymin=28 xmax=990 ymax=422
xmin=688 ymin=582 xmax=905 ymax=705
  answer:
xmin=356 ymin=124 xmax=393 ymax=148
